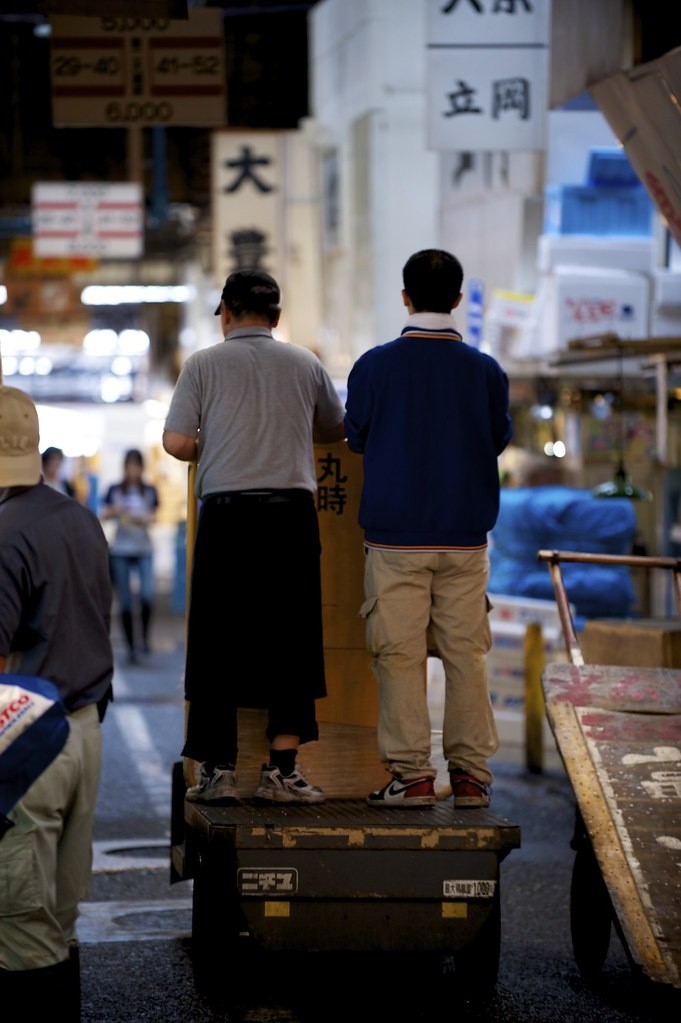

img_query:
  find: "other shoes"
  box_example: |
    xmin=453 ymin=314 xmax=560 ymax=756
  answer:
xmin=128 ymin=651 xmax=138 ymax=666
xmin=137 ymin=638 xmax=149 ymax=654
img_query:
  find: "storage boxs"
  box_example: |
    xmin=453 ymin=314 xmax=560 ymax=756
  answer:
xmin=486 ymin=594 xmax=681 ymax=712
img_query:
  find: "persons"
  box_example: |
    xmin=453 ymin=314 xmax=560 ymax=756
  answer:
xmin=1 ymin=384 xmax=159 ymax=1023
xmin=162 ymin=269 xmax=346 ymax=799
xmin=344 ymin=249 xmax=514 ymax=808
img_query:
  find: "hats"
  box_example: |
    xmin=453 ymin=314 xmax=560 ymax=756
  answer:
xmin=215 ymin=268 xmax=280 ymax=316
xmin=0 ymin=386 xmax=42 ymax=488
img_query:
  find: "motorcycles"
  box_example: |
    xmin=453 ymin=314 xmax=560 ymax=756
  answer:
xmin=165 ymin=437 xmax=526 ymax=1004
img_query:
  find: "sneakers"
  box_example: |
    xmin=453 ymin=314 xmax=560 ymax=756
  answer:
xmin=185 ymin=761 xmax=240 ymax=802
xmin=450 ymin=768 xmax=493 ymax=808
xmin=366 ymin=773 xmax=436 ymax=807
xmin=254 ymin=763 xmax=326 ymax=804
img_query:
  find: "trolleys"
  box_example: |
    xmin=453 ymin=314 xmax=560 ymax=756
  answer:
xmin=540 ymin=545 xmax=681 ymax=990
